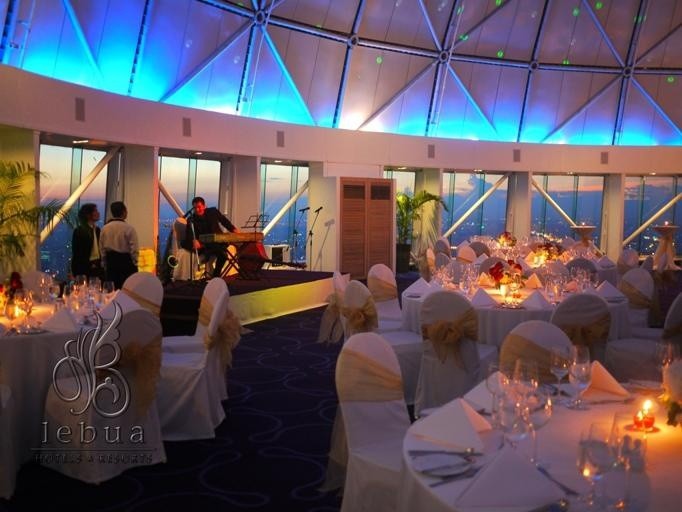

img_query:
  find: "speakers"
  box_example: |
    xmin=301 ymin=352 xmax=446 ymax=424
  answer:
xmin=272 ymin=247 xmax=283 ymax=266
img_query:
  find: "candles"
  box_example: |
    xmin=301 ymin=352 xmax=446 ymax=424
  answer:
xmin=664 ymin=220 xmax=668 ymax=227
xmin=582 ymin=222 xmax=585 ymax=226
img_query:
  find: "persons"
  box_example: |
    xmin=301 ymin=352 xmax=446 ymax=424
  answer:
xmin=185 ymin=197 xmax=240 ymax=277
xmin=69 ymin=202 xmax=102 ymax=281
xmin=98 ymin=202 xmax=141 ymax=291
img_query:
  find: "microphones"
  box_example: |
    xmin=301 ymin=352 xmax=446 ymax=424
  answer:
xmin=299 ymin=207 xmax=310 ymax=212
xmin=183 ymin=206 xmax=195 ymax=218
xmin=314 ymin=206 xmax=324 ymax=214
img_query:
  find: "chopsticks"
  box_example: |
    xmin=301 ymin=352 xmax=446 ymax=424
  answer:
xmin=408 ymin=449 xmax=487 ymax=455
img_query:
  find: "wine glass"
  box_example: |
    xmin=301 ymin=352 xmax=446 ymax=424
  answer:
xmin=569 ymin=344 xmax=590 ymax=409
xmin=521 ymin=387 xmax=551 ymax=465
xmin=549 ymin=347 xmax=570 ymax=405
xmin=0 ymin=271 xmax=114 ymax=336
xmin=492 ymin=385 xmax=526 ymax=451
xmin=487 ymin=362 xmax=510 ymax=430
xmin=513 ymin=359 xmax=540 ymax=429
xmin=576 ymin=412 xmax=647 ymax=512
xmin=472 ymin=236 xmax=602 ymax=263
xmin=443 ymin=263 xmax=599 ymax=304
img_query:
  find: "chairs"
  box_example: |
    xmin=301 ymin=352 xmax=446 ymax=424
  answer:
xmin=171 ymin=218 xmax=239 ymax=280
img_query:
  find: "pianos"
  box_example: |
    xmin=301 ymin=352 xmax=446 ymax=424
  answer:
xmin=198 ymin=232 xmax=263 ymax=243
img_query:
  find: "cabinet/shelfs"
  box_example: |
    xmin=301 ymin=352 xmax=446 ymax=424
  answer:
xmin=305 ymin=177 xmax=396 ymax=280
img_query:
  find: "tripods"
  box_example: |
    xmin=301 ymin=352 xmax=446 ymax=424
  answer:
xmin=245 ymin=227 xmax=270 ymax=278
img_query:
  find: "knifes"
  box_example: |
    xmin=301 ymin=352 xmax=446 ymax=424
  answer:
xmin=415 ymin=462 xmax=486 ymax=486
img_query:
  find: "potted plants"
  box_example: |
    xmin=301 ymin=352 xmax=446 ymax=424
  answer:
xmin=394 ymin=190 xmax=450 ymax=274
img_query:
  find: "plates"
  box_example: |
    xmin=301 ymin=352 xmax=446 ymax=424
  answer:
xmin=411 ymin=454 xmax=470 ymax=476
